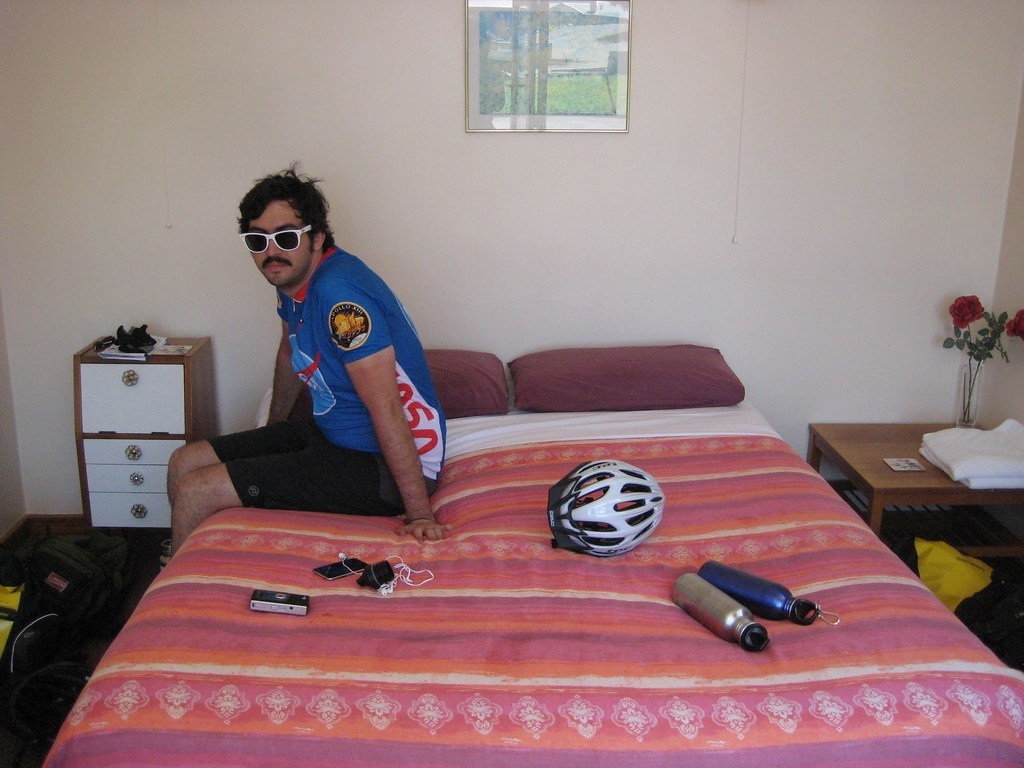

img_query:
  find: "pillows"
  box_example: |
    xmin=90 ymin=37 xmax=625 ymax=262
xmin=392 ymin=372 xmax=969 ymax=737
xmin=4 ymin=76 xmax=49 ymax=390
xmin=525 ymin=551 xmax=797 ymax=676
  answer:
xmin=286 ymin=348 xmax=514 ymax=425
xmin=507 ymin=343 xmax=749 ymax=414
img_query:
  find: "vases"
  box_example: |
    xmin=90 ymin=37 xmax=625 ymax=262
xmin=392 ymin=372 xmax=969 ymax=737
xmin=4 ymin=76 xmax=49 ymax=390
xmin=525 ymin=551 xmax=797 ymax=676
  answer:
xmin=956 ymin=365 xmax=986 ymax=426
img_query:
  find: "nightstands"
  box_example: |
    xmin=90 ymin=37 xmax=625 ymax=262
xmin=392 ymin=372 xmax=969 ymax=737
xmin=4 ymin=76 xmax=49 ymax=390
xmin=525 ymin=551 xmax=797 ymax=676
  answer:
xmin=806 ymin=423 xmax=1023 ymax=557
xmin=73 ymin=336 xmax=216 ymax=532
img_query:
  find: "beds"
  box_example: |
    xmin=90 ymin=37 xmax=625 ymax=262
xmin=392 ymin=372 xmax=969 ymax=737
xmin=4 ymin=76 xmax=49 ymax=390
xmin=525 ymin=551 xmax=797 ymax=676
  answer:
xmin=40 ymin=391 xmax=1023 ymax=767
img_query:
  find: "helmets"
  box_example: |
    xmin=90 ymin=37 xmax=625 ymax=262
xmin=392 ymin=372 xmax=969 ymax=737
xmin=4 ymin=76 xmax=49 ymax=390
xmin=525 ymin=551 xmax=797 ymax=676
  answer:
xmin=546 ymin=459 xmax=665 ymax=557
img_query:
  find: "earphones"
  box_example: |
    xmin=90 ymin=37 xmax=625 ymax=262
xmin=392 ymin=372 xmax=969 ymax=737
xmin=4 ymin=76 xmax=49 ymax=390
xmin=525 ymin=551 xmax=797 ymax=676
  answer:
xmin=338 ymin=553 xmax=347 ymax=560
xmin=380 ymin=587 xmax=387 ymax=596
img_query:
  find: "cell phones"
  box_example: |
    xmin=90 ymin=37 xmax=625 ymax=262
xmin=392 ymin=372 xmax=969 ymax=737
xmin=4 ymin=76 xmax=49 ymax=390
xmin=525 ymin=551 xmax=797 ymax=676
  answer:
xmin=314 ymin=558 xmax=368 ymax=581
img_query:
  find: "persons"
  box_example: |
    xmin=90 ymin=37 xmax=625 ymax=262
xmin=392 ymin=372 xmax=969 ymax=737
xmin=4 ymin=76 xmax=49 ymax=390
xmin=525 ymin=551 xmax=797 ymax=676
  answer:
xmin=167 ymin=174 xmax=454 ymax=558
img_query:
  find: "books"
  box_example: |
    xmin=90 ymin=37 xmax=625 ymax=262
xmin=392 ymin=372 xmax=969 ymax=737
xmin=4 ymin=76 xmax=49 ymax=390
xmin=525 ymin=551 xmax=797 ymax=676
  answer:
xmin=99 ymin=336 xmax=167 ymax=361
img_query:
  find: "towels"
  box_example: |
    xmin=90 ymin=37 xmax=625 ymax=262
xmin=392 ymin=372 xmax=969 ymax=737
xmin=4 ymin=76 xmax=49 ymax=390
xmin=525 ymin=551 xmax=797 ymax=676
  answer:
xmin=918 ymin=416 xmax=1024 ymax=490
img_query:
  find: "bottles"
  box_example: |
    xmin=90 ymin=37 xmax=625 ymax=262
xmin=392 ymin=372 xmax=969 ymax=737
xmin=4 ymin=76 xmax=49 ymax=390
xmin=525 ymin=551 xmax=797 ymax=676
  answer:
xmin=671 ymin=572 xmax=770 ymax=651
xmin=697 ymin=560 xmax=840 ymax=626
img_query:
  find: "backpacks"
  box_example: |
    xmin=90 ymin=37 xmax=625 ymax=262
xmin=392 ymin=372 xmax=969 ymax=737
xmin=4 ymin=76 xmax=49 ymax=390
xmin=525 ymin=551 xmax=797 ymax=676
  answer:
xmin=20 ymin=532 xmax=127 ymax=652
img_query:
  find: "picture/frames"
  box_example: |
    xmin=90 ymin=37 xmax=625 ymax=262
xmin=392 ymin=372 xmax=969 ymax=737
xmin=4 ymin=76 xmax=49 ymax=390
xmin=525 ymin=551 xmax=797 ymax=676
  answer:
xmin=461 ymin=2 xmax=633 ymax=135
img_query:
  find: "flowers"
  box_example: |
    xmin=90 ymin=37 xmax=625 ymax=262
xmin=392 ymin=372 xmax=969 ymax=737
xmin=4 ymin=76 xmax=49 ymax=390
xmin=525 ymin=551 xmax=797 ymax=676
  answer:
xmin=942 ymin=294 xmax=1024 ymax=363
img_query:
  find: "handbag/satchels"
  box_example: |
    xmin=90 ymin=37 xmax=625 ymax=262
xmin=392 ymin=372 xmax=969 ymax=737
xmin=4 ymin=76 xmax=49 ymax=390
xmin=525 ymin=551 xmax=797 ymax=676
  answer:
xmin=892 ymin=536 xmax=1024 ymax=672
xmin=0 ymin=562 xmax=59 ymax=678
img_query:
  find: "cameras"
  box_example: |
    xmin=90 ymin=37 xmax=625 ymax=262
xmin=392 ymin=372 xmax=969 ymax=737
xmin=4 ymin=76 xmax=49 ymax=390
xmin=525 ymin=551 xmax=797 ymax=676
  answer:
xmin=250 ymin=590 xmax=309 ymax=616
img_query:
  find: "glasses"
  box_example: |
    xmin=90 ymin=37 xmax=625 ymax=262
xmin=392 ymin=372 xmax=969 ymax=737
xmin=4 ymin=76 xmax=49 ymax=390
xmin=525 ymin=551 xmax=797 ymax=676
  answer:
xmin=239 ymin=224 xmax=312 ymax=253
